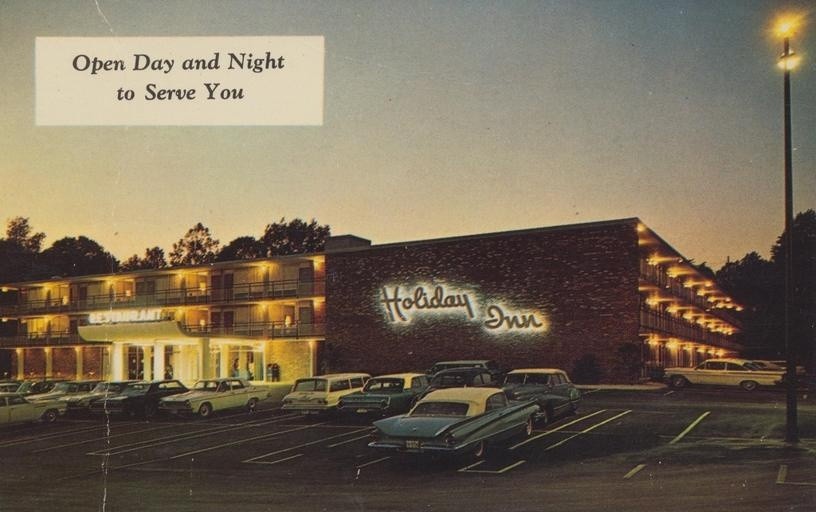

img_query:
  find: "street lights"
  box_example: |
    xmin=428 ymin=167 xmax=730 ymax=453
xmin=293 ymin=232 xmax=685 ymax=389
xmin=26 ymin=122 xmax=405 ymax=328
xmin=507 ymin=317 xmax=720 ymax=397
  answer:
xmin=773 ymin=10 xmax=805 ymax=442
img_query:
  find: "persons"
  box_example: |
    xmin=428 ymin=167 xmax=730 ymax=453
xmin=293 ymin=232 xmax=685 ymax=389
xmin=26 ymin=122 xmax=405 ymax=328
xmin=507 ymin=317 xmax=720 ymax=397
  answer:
xmin=231 ymin=358 xmax=239 ymax=377
xmin=271 ymin=363 xmax=280 ymax=383
xmin=266 ymin=363 xmax=271 ymax=384
xmin=128 ymin=364 xmax=173 ymax=381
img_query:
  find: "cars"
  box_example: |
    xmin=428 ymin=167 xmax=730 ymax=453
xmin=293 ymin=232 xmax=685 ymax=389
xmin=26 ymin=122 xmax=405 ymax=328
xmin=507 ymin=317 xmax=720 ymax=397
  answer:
xmin=0 ymin=377 xmax=272 ymax=425
xmin=663 ymin=357 xmax=806 ymax=391
xmin=280 ymin=360 xmax=581 ymax=457
